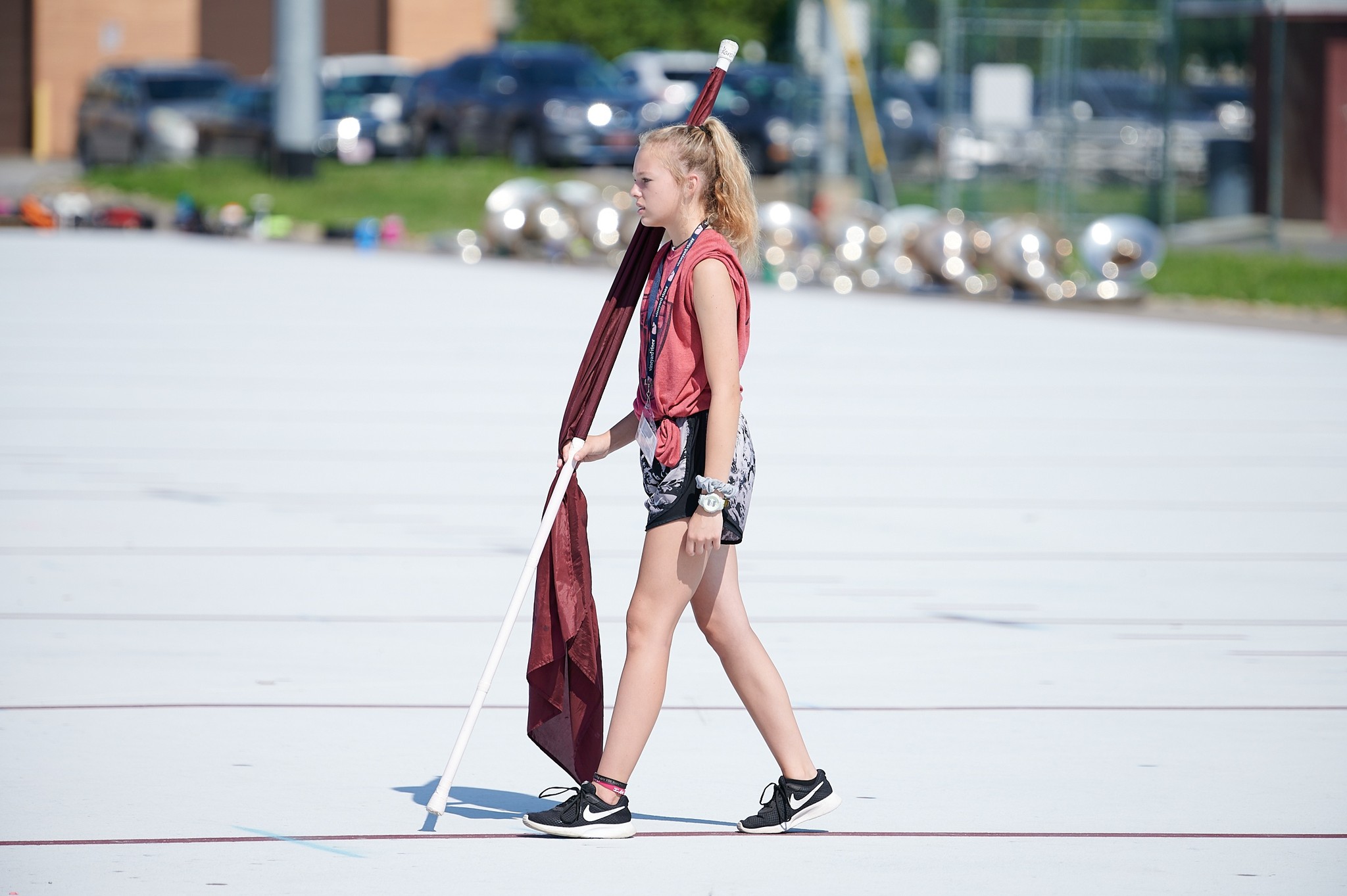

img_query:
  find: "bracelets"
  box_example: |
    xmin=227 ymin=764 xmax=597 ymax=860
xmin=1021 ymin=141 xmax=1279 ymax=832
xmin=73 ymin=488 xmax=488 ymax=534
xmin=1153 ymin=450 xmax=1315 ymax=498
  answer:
xmin=695 ymin=474 xmax=733 ymax=495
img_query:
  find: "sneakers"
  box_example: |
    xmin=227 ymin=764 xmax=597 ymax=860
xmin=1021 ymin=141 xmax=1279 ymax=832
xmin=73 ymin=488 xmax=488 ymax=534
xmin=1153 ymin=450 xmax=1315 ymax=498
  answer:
xmin=737 ymin=769 xmax=841 ymax=834
xmin=522 ymin=780 xmax=637 ymax=838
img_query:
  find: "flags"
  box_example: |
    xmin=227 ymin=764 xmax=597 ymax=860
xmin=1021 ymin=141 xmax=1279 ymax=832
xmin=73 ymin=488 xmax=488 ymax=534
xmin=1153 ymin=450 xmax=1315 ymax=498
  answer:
xmin=527 ymin=66 xmax=726 ymax=786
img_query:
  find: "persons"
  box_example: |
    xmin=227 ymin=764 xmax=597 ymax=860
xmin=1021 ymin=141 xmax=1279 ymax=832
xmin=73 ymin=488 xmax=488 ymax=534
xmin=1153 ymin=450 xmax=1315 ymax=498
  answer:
xmin=521 ymin=114 xmax=838 ymax=837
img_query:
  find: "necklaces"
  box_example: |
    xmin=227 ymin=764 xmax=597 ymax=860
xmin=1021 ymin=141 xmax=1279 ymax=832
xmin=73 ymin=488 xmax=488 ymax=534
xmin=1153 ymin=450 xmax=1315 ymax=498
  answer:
xmin=671 ymin=238 xmax=691 ymax=251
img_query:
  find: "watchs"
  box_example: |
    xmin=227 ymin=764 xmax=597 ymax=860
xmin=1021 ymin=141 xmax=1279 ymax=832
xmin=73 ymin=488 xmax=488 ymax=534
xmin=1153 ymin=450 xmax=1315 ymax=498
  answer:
xmin=698 ymin=493 xmax=728 ymax=514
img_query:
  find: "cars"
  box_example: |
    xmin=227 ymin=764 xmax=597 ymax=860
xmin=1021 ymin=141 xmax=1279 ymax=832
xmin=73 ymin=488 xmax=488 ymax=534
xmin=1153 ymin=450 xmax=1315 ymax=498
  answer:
xmin=70 ymin=39 xmax=1259 ymax=189
